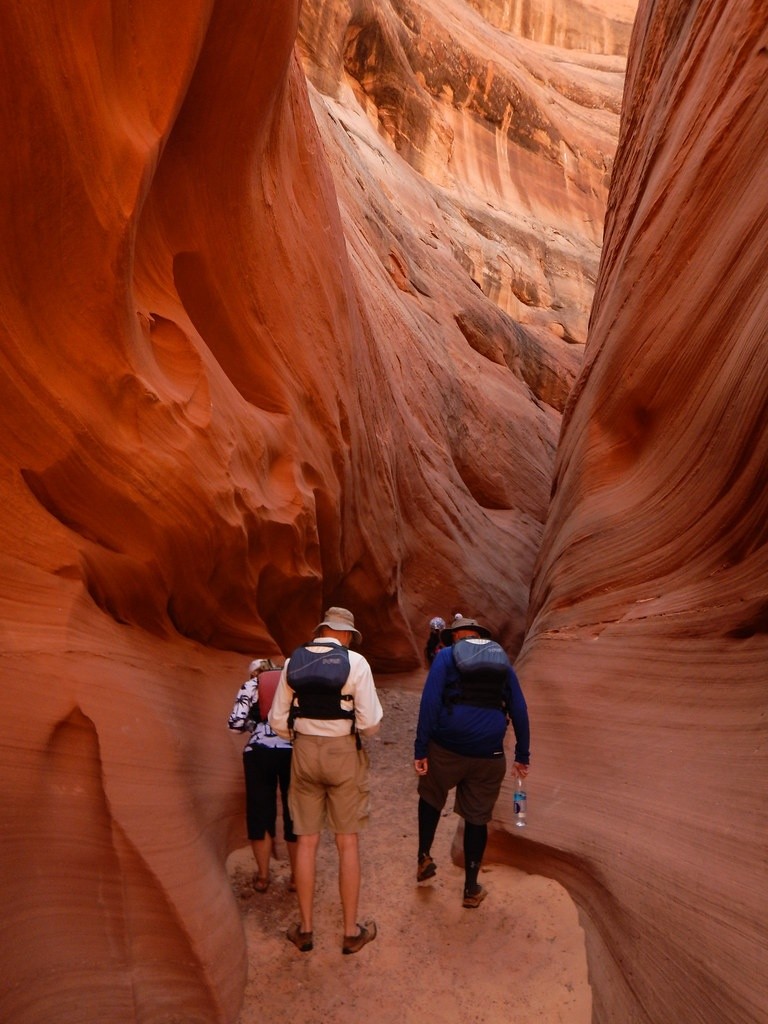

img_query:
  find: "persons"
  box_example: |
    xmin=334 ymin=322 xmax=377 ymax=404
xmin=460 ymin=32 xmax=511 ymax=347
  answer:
xmin=423 ymin=617 xmax=446 ymax=670
xmin=414 ymin=618 xmax=531 ymax=908
xmin=226 ymin=658 xmax=298 ymax=895
xmin=269 ymin=607 xmax=384 ymax=955
xmin=454 ymin=613 xmax=463 ymax=621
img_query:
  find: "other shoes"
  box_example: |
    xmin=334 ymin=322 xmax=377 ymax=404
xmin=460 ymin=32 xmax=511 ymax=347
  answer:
xmin=417 ymin=853 xmax=437 ymax=882
xmin=252 ymin=872 xmax=269 ymax=892
xmin=287 ymin=922 xmax=313 ymax=951
xmin=464 ymin=882 xmax=484 ymax=908
xmin=343 ymin=921 xmax=377 ymax=954
xmin=288 ymin=875 xmax=297 ymax=892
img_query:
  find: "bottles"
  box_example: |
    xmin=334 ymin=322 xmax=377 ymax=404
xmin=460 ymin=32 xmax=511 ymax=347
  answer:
xmin=512 ymin=768 xmax=528 ymax=826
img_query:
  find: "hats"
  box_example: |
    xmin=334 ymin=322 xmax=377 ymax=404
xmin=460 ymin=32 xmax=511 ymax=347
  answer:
xmin=440 ymin=619 xmax=490 ymax=646
xmin=312 ymin=607 xmax=361 ymax=644
xmin=430 ymin=617 xmax=446 ymax=633
xmin=455 ymin=614 xmax=463 ymax=620
xmin=249 ymin=659 xmax=276 ymax=679
